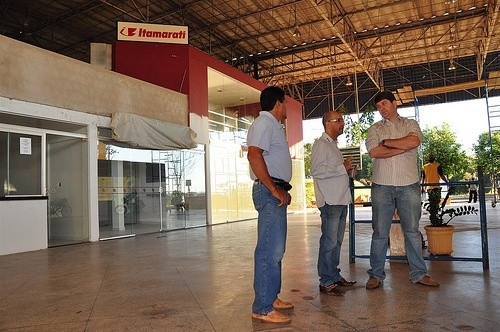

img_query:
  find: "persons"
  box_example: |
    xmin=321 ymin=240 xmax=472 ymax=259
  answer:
xmin=468 ymin=177 xmax=477 ymax=203
xmin=247 ymin=87 xmax=295 ymax=326
xmin=421 ymin=154 xmax=449 ymax=212
xmin=364 ymin=91 xmax=441 ymax=290
xmin=310 ymin=111 xmax=356 ymax=296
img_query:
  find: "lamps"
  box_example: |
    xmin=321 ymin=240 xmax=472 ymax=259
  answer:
xmin=448 ymin=58 xmax=456 ymax=71
xmin=346 ymin=73 xmax=353 ymax=86
xmin=292 ymin=3 xmax=302 ymax=38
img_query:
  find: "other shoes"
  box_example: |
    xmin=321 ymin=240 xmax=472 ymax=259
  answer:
xmin=366 ymin=277 xmax=380 ymax=289
xmin=273 ymin=298 xmax=294 ymax=309
xmin=252 ymin=309 xmax=292 ymax=323
xmin=418 ymin=275 xmax=440 ymax=286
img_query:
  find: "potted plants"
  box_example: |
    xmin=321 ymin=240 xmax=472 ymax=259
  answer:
xmin=421 ymin=186 xmax=479 ymax=256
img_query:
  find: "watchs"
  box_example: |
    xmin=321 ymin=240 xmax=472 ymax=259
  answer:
xmin=381 ymin=138 xmax=387 ymax=147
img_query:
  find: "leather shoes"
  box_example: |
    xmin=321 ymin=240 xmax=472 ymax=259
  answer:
xmin=318 ymin=282 xmax=346 ymax=296
xmin=336 ymin=277 xmax=357 ymax=286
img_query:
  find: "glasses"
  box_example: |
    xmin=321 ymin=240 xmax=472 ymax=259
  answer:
xmin=328 ymin=118 xmax=344 ymax=122
